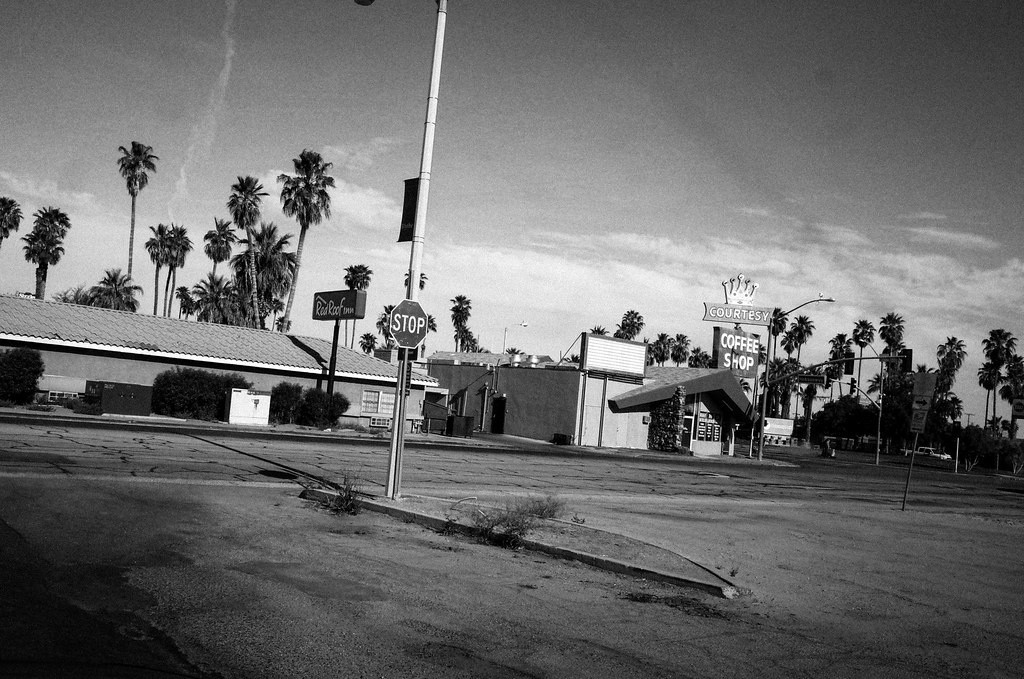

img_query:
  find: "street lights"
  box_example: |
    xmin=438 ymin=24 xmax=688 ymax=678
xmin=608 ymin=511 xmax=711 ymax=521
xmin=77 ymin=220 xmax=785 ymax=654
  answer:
xmin=858 ymin=338 xmax=884 ymax=466
xmin=503 ymin=321 xmax=528 ymax=353
xmin=757 ymin=292 xmax=836 ymax=458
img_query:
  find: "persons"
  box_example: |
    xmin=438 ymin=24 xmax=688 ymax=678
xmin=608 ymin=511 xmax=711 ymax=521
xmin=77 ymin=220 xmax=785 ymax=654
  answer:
xmin=824 ymin=439 xmax=830 ymax=458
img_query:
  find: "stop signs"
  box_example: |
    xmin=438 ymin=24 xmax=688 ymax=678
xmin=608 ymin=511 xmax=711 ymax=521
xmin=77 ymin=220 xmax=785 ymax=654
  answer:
xmin=389 ymin=300 xmax=428 ymax=349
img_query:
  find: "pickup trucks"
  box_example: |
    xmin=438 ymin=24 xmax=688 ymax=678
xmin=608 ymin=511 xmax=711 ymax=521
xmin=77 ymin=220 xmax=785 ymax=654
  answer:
xmin=900 ymin=446 xmax=952 ymax=460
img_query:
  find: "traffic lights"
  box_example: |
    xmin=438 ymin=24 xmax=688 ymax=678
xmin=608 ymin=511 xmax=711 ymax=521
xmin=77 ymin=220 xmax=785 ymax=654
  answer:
xmin=825 ymin=374 xmax=832 ymax=390
xmin=850 ymin=377 xmax=857 ymax=395
